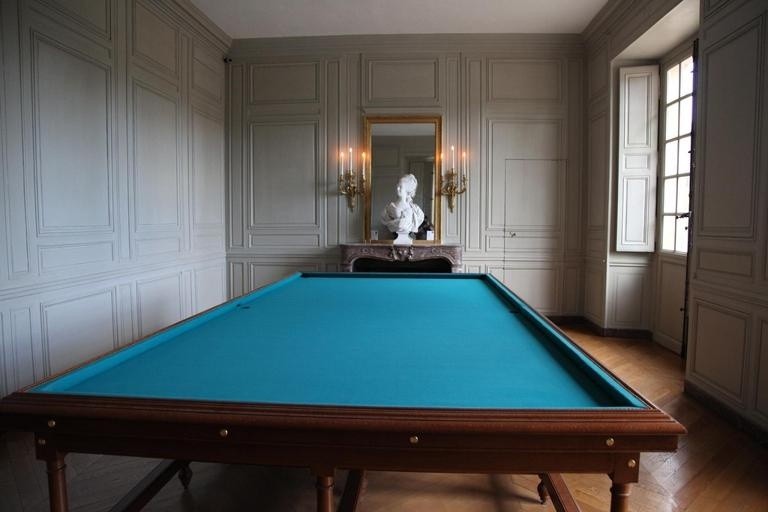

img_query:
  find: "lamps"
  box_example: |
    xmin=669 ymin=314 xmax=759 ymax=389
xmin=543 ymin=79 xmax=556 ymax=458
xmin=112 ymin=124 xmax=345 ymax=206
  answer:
xmin=339 ymin=148 xmax=366 ymax=212
xmin=440 ymin=145 xmax=468 ymax=213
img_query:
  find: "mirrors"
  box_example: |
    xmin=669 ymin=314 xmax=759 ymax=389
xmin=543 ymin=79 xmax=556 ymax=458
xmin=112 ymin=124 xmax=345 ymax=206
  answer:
xmin=363 ymin=114 xmax=443 ymax=244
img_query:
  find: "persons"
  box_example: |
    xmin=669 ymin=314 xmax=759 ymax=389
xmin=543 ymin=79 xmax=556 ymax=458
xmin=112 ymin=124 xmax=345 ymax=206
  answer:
xmin=380 ymin=173 xmax=424 ymax=247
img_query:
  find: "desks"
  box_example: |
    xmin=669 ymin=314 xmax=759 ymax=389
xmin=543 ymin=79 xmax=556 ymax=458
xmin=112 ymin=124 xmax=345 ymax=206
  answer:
xmin=339 ymin=242 xmax=462 ymax=273
xmin=0 ymin=272 xmax=686 ymax=512
